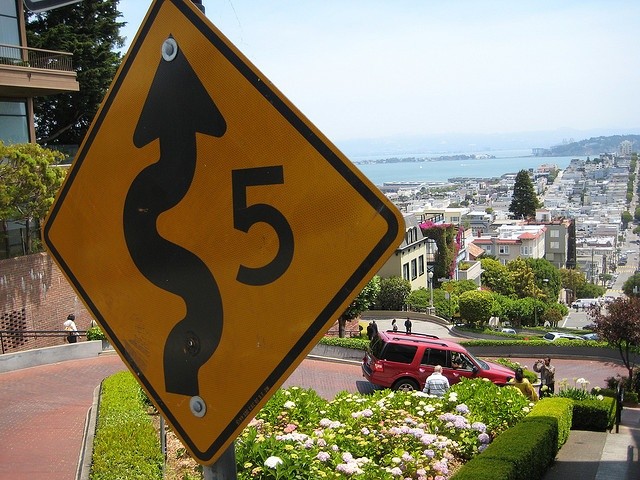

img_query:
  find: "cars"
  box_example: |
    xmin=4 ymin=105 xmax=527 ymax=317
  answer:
xmin=583 ymin=333 xmax=596 ymax=340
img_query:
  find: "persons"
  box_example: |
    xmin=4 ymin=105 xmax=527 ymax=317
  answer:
xmin=392 ymin=319 xmax=398 ymax=330
xmin=405 ymin=317 xmax=411 ymax=333
xmin=372 ymin=320 xmax=378 ymax=338
xmin=508 ymin=368 xmax=533 ymax=399
xmin=366 ymin=322 xmax=375 ymax=340
xmin=63 ymin=314 xmax=82 ymax=343
xmin=422 ymin=365 xmax=450 ymax=395
xmin=451 ymin=353 xmax=464 ymax=368
xmin=533 ymin=356 xmax=555 ymax=400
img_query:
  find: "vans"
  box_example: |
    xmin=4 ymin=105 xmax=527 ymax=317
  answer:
xmin=494 ymin=328 xmax=516 ymax=335
xmin=571 ymin=298 xmax=597 ymax=308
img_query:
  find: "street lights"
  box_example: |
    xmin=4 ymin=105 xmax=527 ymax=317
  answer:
xmin=428 ymin=271 xmax=433 ymax=307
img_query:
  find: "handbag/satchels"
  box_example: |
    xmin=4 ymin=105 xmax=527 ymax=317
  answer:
xmin=64 ymin=324 xmax=74 ymax=335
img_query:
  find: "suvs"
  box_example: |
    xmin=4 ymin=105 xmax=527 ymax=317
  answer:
xmin=361 ymin=330 xmax=516 ymax=392
xmin=542 ymin=332 xmax=584 ymax=342
